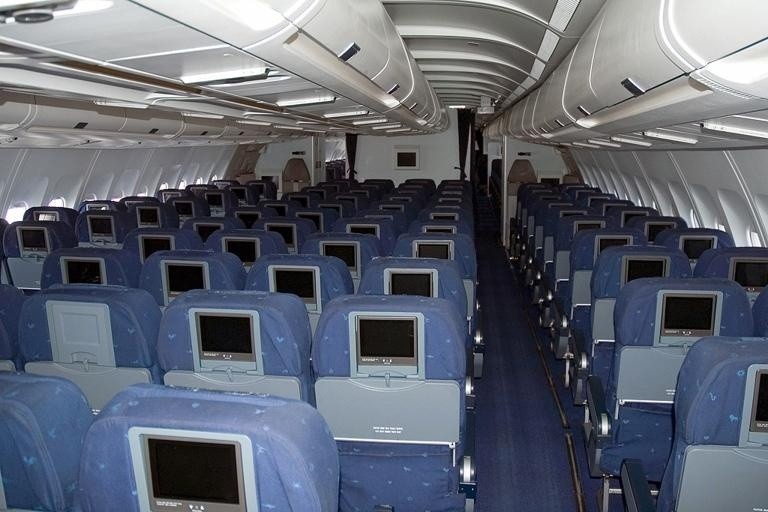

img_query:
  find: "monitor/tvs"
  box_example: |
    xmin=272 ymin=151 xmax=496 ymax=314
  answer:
xmin=349 ymin=311 xmax=425 ymax=380
xmin=187 ymin=308 xmax=264 ymax=374
xmin=395 ymin=150 xmax=419 ymax=168
xmin=749 ymin=369 xmax=768 ymax=432
xmin=143 ymin=435 xmax=247 ymax=512
xmin=653 ymin=289 xmax=724 ymax=348
